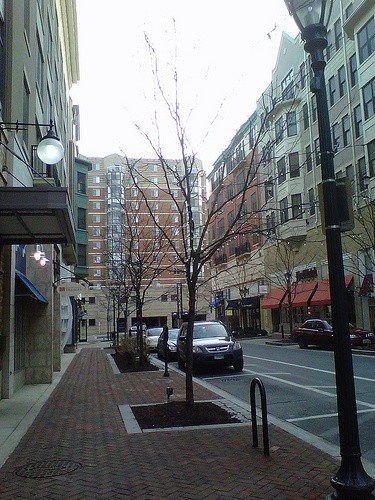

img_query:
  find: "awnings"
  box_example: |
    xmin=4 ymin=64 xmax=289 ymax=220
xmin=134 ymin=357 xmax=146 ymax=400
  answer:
xmin=15 ymin=270 xmax=50 ymax=305
xmin=209 ymin=299 xmax=224 ymax=305
xmin=225 ymin=294 xmax=263 ymax=309
xmin=261 ymin=275 xmax=354 ymax=309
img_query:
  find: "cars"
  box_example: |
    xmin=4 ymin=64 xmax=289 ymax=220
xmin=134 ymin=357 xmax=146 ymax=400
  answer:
xmin=157 ymin=329 xmax=183 ymax=361
xmin=146 ymin=327 xmax=168 ymax=349
xmin=131 ymin=322 xmax=147 ymax=331
xmin=293 ymin=318 xmax=374 ymax=350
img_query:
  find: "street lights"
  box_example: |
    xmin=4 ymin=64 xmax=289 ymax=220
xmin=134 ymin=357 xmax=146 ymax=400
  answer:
xmin=286 ymin=0 xmax=374 ymax=500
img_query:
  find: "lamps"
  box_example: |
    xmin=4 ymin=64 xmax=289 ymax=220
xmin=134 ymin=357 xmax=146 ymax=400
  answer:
xmin=0 ymin=119 xmax=64 ymax=164
xmin=30 ymin=245 xmax=50 ymax=267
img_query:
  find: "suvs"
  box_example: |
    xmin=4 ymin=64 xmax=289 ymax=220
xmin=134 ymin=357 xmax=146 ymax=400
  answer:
xmin=176 ymin=321 xmax=245 ymax=371
